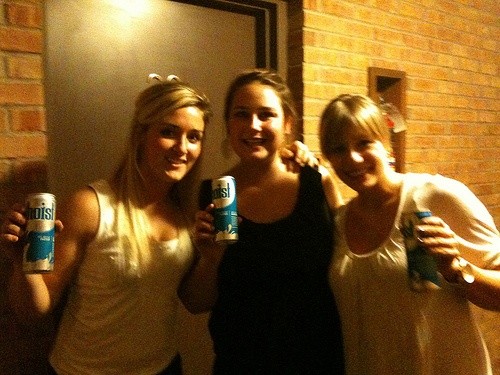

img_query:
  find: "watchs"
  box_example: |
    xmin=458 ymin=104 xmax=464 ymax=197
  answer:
xmin=445 ymin=255 xmax=475 ymax=289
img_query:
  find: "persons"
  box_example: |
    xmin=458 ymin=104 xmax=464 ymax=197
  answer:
xmin=310 ymin=93 xmax=500 ymax=375
xmin=0 ymin=81 xmax=319 ymax=375
xmin=178 ymin=69 xmax=343 ymax=375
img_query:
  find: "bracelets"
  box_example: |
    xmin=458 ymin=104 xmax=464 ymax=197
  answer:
xmin=312 ymin=158 xmax=320 ymax=170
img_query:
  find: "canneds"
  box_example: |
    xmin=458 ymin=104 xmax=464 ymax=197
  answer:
xmin=23 ymin=192 xmax=56 ymax=274
xmin=212 ymin=176 xmax=239 ymax=243
xmin=401 ymin=207 xmax=440 ymax=293
